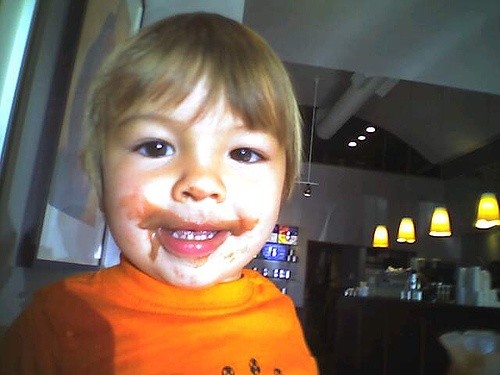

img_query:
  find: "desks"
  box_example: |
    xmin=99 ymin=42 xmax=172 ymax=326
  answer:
xmin=333 ymin=294 xmax=500 ymax=375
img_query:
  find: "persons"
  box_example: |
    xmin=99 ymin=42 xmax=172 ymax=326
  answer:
xmin=0 ymin=11 xmax=318 ymax=375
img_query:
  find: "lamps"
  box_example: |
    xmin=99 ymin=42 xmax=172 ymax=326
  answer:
xmin=303 ymin=76 xmax=322 ymax=197
xmin=396 ymin=217 xmax=415 ymax=243
xmin=372 ymin=224 xmax=389 ymax=248
xmin=428 ymin=207 xmax=452 ymax=237
xmin=474 ymin=192 xmax=500 ymax=229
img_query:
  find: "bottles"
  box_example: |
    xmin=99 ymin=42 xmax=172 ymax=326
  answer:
xmin=409 ymin=274 xmax=418 ymax=301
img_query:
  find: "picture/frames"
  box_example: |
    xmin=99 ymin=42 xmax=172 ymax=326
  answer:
xmin=16 ymin=0 xmax=148 ymax=272
